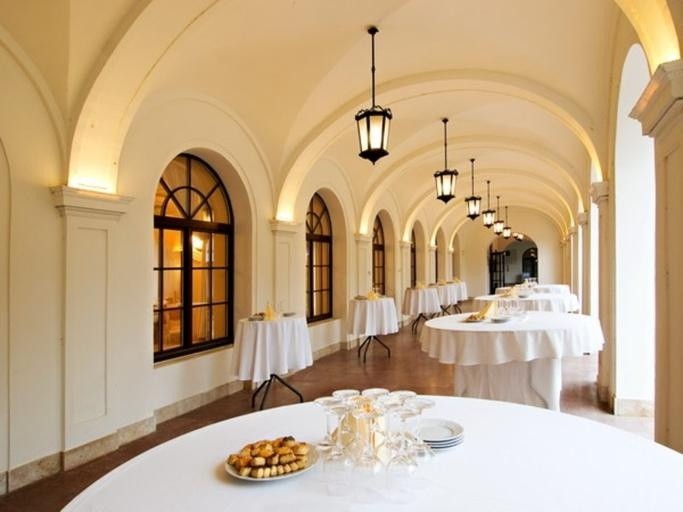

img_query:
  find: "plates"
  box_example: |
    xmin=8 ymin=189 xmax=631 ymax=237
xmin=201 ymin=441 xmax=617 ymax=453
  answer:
xmin=491 ymin=316 xmax=510 ymax=323
xmin=464 ymin=319 xmax=482 ymax=322
xmin=420 ymin=419 xmax=463 ymax=448
xmin=518 ymin=294 xmax=528 ymax=298
xmin=225 ymin=443 xmax=319 ymax=482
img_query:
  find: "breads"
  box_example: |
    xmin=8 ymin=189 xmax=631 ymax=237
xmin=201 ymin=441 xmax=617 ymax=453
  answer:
xmin=228 ymin=436 xmax=310 ymax=478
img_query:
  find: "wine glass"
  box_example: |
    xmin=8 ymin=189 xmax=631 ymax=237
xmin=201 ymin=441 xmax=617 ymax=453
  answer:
xmin=312 ymin=386 xmax=441 ymax=504
xmin=527 ymin=278 xmax=537 ymax=288
xmin=497 ymin=298 xmax=528 ymax=320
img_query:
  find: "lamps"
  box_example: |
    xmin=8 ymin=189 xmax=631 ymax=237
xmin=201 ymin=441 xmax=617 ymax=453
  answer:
xmin=482 ymin=180 xmax=496 ymax=230
xmin=434 ymin=116 xmax=459 ymax=206
xmin=464 ymin=157 xmax=481 ymax=223
xmin=353 ymin=21 xmax=394 ymax=168
xmin=503 ymin=205 xmax=511 ymax=239
xmin=494 ymin=195 xmax=504 ymax=237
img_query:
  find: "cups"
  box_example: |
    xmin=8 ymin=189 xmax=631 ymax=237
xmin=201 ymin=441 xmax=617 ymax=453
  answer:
xmin=264 ymin=301 xmax=282 ymax=320
xmin=366 ymin=287 xmax=379 ymax=300
